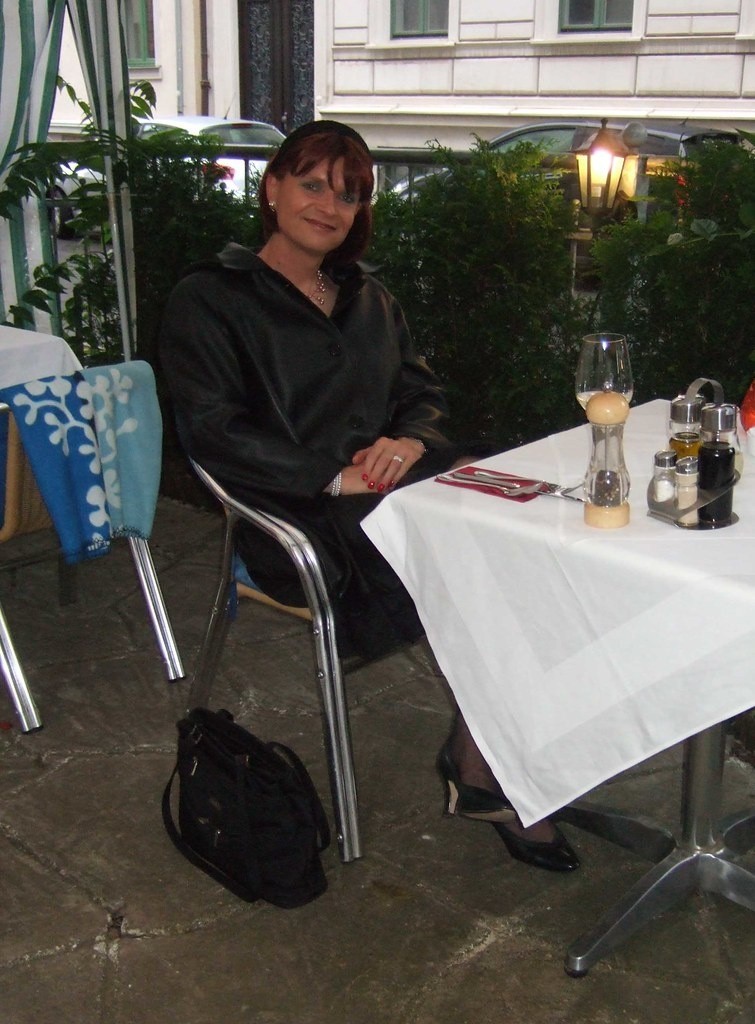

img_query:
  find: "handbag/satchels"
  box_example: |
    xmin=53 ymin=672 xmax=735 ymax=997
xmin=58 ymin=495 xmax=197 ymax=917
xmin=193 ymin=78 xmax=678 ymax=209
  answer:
xmin=161 ymin=706 xmax=331 ymax=909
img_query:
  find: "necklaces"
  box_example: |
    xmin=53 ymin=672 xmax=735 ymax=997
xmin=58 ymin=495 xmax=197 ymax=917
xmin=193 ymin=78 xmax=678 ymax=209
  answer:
xmin=307 ymin=269 xmax=329 ymax=306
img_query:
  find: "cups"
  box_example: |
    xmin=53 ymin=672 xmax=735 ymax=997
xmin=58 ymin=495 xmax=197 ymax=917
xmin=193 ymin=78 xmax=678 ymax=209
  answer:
xmin=669 ymin=396 xmax=702 ymax=484
xmin=699 ymin=403 xmax=733 ymax=524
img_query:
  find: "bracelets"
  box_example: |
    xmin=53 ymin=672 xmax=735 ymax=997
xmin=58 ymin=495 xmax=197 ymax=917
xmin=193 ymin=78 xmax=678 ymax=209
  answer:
xmin=331 ymin=472 xmax=342 ymax=496
xmin=415 ymin=439 xmax=428 ymax=453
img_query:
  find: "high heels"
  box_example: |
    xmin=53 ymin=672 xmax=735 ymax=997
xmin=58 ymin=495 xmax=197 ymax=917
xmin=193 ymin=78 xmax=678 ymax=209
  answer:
xmin=438 ymin=744 xmax=516 ymax=822
xmin=492 ymin=817 xmax=580 ymax=873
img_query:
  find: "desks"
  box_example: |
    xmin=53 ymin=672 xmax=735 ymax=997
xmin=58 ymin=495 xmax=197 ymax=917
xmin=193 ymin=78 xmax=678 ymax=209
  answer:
xmin=359 ymin=398 xmax=755 ymax=976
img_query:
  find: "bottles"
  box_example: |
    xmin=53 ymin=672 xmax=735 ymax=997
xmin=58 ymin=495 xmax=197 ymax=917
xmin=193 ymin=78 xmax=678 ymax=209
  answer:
xmin=653 ymin=450 xmax=676 ymax=501
xmin=674 ymin=455 xmax=698 ymax=525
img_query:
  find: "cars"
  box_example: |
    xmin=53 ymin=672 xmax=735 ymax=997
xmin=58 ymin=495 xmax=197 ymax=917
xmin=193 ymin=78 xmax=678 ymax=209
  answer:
xmin=43 ymin=137 xmax=109 ymax=237
xmin=95 ymin=116 xmax=288 ymax=220
xmin=387 ymin=121 xmax=747 ymax=291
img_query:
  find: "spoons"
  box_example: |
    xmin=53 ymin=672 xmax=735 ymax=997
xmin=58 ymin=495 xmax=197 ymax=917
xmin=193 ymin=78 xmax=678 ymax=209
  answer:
xmin=436 ymin=473 xmax=541 ymax=497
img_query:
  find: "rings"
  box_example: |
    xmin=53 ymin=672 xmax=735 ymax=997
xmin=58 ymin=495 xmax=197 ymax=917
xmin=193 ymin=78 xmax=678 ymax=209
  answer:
xmin=393 ymin=456 xmax=403 ymax=463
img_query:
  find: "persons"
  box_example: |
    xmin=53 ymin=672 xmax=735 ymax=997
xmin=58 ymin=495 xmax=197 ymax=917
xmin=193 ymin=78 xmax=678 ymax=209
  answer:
xmin=156 ymin=120 xmax=585 ymax=874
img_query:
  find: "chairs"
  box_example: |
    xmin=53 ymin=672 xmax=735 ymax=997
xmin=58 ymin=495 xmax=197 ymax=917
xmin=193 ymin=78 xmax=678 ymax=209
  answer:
xmin=181 ymin=452 xmax=385 ymax=861
xmin=1 ymin=361 xmax=185 ymax=734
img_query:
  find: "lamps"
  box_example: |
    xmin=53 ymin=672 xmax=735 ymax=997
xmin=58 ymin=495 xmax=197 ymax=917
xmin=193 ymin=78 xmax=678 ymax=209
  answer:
xmin=574 ymin=118 xmax=630 ymax=222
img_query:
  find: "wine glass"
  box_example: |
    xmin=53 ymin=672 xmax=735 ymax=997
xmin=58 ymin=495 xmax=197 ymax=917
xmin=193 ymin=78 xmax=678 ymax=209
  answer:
xmin=577 ymin=334 xmax=634 ymax=470
